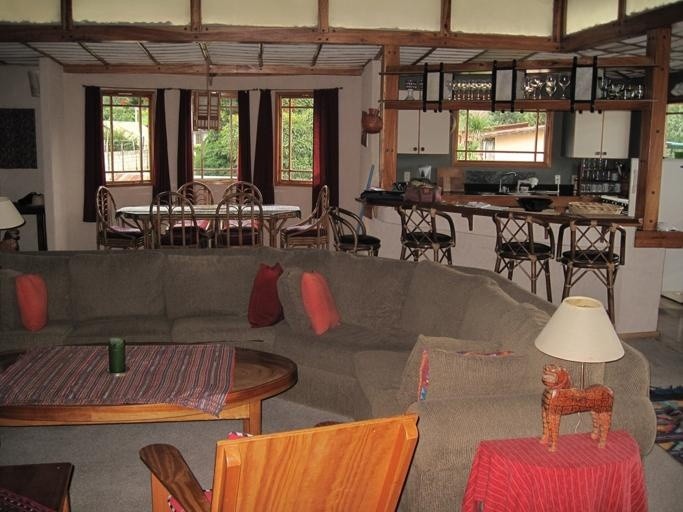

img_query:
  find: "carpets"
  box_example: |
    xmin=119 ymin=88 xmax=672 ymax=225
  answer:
xmin=649 ymin=384 xmax=683 ymax=466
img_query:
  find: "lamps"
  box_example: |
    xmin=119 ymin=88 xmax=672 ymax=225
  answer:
xmin=534 ymin=296 xmax=625 ymax=451
xmin=490 ymin=59 xmax=527 ymax=113
xmin=0 ymin=197 xmax=25 ymax=251
xmin=571 ymin=56 xmax=606 ymax=114
xmin=422 ymin=62 xmax=454 ymax=112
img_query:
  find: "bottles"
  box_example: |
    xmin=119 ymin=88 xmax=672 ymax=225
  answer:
xmin=579 ymin=158 xmax=621 ymax=194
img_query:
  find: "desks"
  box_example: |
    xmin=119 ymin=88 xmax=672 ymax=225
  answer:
xmin=462 ymin=432 xmax=649 ymax=512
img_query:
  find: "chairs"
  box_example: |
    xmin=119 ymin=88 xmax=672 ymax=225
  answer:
xmin=96 ymin=180 xmax=329 ymax=251
xmin=326 ymin=207 xmax=381 ymax=256
xmin=0 ymin=462 xmax=75 ymax=512
xmin=556 ymin=220 xmax=625 ymax=328
xmin=139 ymin=414 xmax=419 ymax=512
xmin=493 ymin=210 xmax=555 ymax=305
xmin=398 ymin=204 xmax=456 ymax=265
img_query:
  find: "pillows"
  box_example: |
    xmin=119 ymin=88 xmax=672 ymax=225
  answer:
xmin=400 ymin=261 xmax=605 ymax=408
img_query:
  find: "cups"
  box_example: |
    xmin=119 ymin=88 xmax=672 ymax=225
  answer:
xmin=108 ymin=338 xmax=126 ymax=377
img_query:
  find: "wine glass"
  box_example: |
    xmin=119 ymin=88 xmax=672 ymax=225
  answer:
xmin=521 ymin=74 xmax=571 ymax=100
xmin=404 ymin=79 xmax=422 ymax=100
xmin=597 ymin=77 xmax=644 ymax=100
xmin=445 ymin=79 xmax=491 ymax=101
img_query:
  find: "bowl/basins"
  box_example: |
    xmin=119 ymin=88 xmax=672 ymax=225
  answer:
xmin=515 ymin=197 xmax=554 ymax=212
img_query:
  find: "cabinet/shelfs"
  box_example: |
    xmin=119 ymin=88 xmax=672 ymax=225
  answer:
xmin=565 ymin=109 xmax=631 ymax=159
xmin=396 ymin=90 xmax=450 ymax=155
